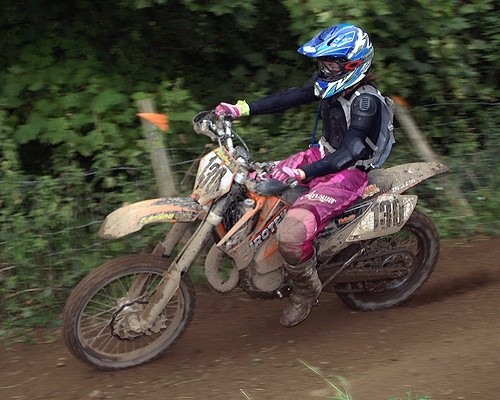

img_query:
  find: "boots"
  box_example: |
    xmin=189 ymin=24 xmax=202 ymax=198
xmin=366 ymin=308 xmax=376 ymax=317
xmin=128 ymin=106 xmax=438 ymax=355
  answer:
xmin=278 ymin=245 xmax=323 ymax=327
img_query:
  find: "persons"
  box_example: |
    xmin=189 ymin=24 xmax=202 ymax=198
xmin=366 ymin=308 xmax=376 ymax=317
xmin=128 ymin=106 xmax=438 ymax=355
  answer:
xmin=214 ymin=23 xmax=382 ymax=329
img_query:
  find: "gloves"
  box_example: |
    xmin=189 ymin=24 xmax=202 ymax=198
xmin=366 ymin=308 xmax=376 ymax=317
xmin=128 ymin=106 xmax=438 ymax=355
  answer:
xmin=271 ymin=166 xmax=302 ymax=186
xmin=214 ymin=102 xmax=242 ymax=118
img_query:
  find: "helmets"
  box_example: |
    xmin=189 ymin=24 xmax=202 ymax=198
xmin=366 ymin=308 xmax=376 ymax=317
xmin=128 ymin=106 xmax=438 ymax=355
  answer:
xmin=297 ymin=23 xmax=374 ymax=100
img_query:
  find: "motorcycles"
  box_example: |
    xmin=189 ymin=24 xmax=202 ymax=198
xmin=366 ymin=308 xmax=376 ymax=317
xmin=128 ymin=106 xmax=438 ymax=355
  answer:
xmin=58 ymin=108 xmax=450 ymax=370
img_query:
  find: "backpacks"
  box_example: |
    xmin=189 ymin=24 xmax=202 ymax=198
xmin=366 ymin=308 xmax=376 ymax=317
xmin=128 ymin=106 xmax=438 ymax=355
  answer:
xmin=338 ymin=85 xmax=397 ymax=170
